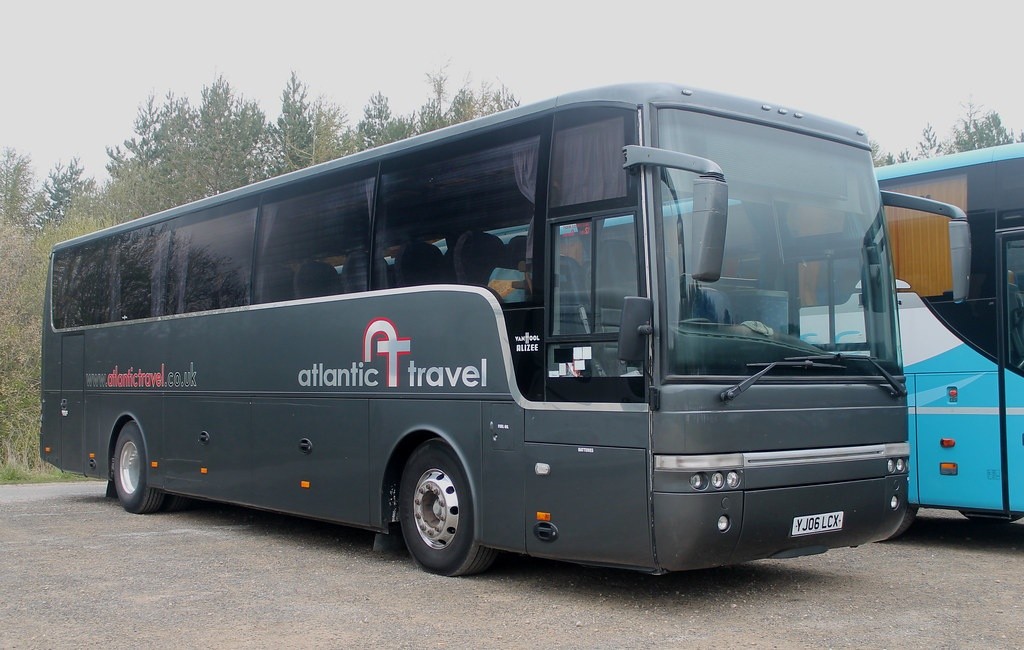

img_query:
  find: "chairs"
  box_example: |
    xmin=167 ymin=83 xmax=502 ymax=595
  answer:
xmin=247 ymin=230 xmax=528 ymax=309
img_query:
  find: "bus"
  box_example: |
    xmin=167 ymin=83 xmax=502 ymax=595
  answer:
xmin=39 ymin=82 xmax=972 ymax=578
xmin=333 ymin=143 xmax=1024 ymax=543
